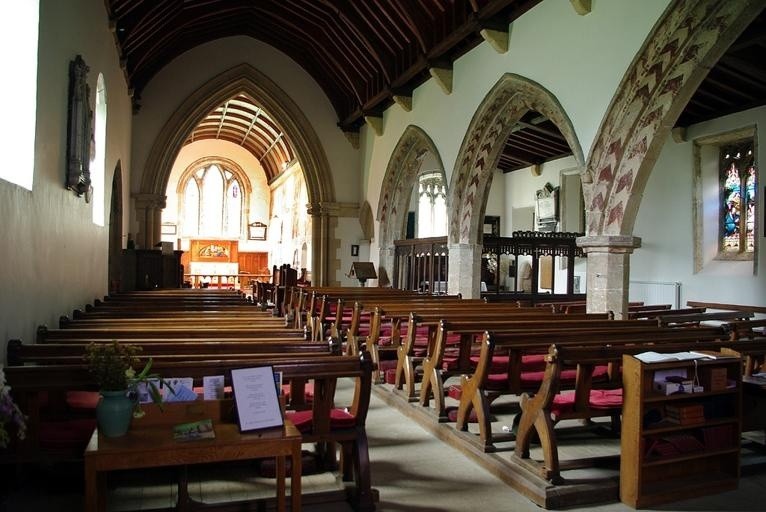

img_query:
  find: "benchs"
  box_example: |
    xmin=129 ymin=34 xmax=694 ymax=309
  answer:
xmin=0 ymin=290 xmax=370 ymax=512
xmin=253 ymin=266 xmax=756 ymax=509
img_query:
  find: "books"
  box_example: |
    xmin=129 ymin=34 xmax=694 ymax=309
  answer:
xmin=742 ymin=430 xmax=766 ymax=444
xmin=633 ymin=350 xmax=742 ymax=457
xmin=171 ymin=417 xmax=216 ymax=443
xmin=741 ymin=374 xmax=766 ymax=385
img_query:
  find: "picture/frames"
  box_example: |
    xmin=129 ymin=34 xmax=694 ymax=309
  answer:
xmin=536 ymin=185 xmax=558 ymax=233
xmin=68 ymin=53 xmax=91 ymax=200
xmin=248 ymin=222 xmax=267 ymax=240
